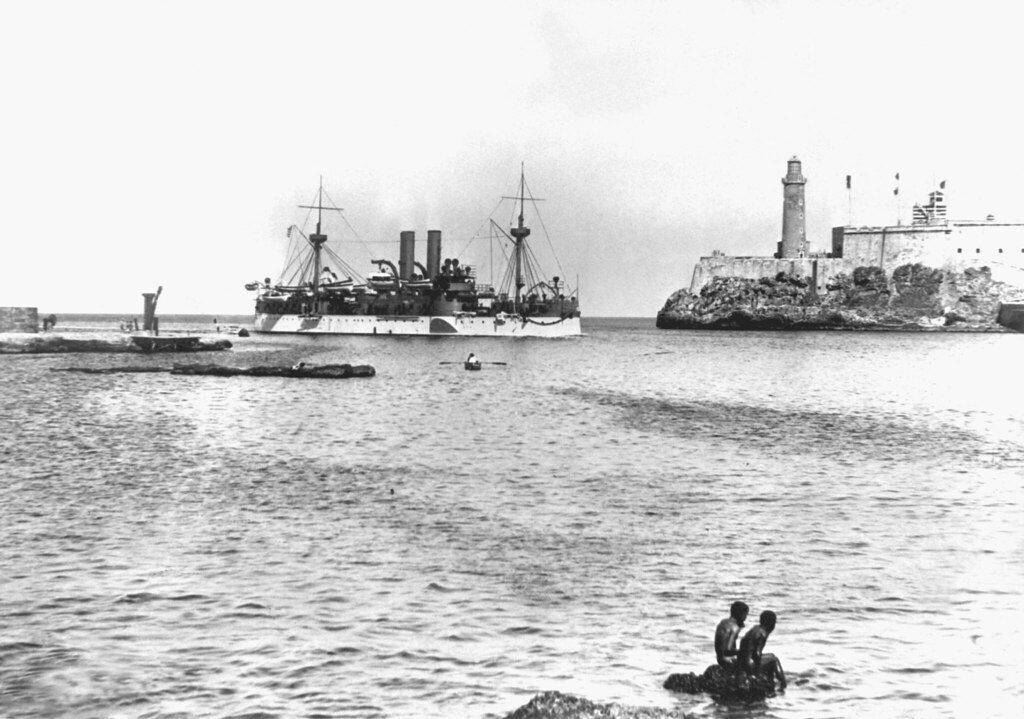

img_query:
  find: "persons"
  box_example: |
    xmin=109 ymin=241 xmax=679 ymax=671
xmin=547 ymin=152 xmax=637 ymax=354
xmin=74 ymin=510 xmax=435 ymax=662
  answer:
xmin=716 ymin=601 xmax=786 ymax=687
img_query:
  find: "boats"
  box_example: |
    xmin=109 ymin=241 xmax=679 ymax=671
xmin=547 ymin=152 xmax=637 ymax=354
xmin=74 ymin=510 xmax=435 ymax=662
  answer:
xmin=244 ymin=162 xmax=581 ymax=337
xmin=132 ymin=336 xmax=202 ymax=352
xmin=465 ymin=362 xmax=482 ymax=371
